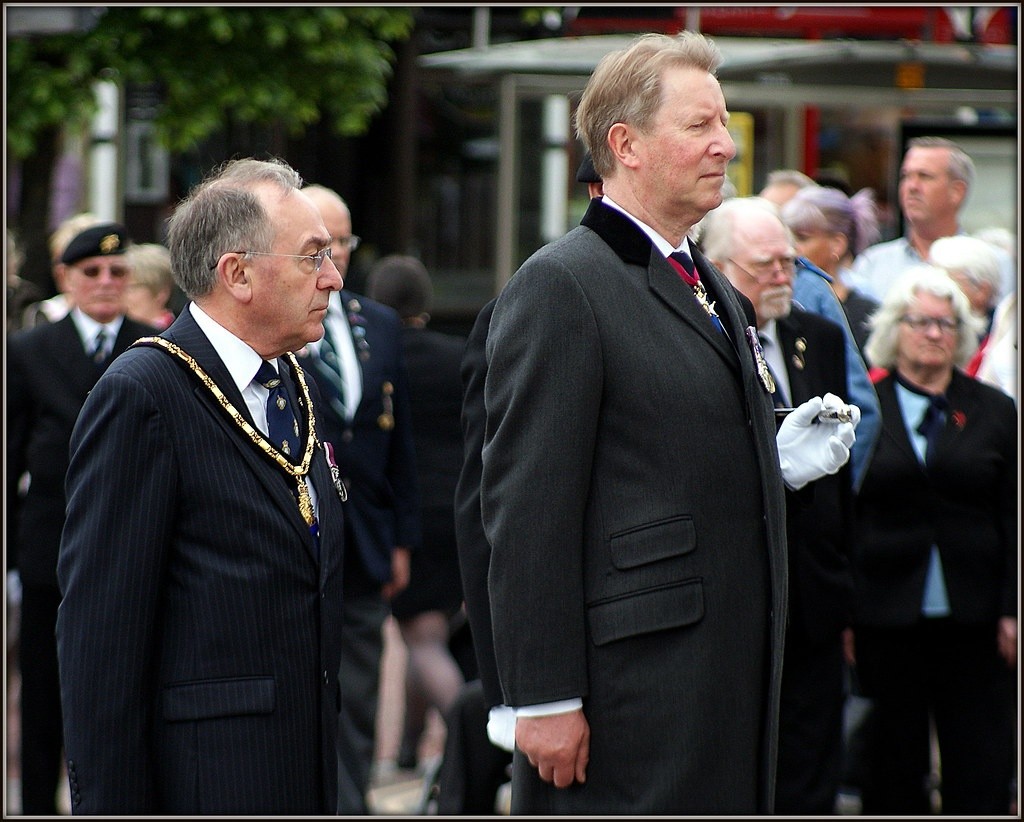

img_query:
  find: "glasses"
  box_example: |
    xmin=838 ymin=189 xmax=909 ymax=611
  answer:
xmin=209 ymin=247 xmax=332 ymax=270
xmin=898 ymin=311 xmax=961 ymax=333
xmin=73 ymin=264 xmax=129 ymax=277
xmin=726 ymin=256 xmax=796 ymax=287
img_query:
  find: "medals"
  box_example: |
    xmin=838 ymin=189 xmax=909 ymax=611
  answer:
xmin=693 ymin=288 xmax=720 ymax=319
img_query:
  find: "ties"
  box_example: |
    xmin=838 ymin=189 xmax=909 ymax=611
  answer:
xmin=759 ymin=336 xmax=791 ymax=434
xmin=669 ymin=249 xmax=722 ymax=332
xmin=318 ymin=308 xmax=346 ymax=424
xmin=253 ymin=361 xmax=319 ymax=544
xmin=93 ymin=331 xmax=107 ymax=365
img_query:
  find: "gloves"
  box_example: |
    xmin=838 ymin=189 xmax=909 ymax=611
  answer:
xmin=775 ymin=392 xmax=861 ymax=491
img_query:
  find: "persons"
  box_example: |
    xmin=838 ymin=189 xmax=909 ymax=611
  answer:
xmin=296 ymin=180 xmax=512 ymax=821
xmin=1 ymin=213 xmax=174 ymax=818
xmin=54 ymin=149 xmax=348 ymax=822
xmin=453 ymin=25 xmax=1023 ymax=821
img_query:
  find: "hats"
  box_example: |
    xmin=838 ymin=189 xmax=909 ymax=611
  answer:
xmin=60 ymin=222 xmax=127 ymax=264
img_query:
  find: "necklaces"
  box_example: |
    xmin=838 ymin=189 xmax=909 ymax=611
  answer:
xmin=126 ymin=337 xmax=319 ymax=526
